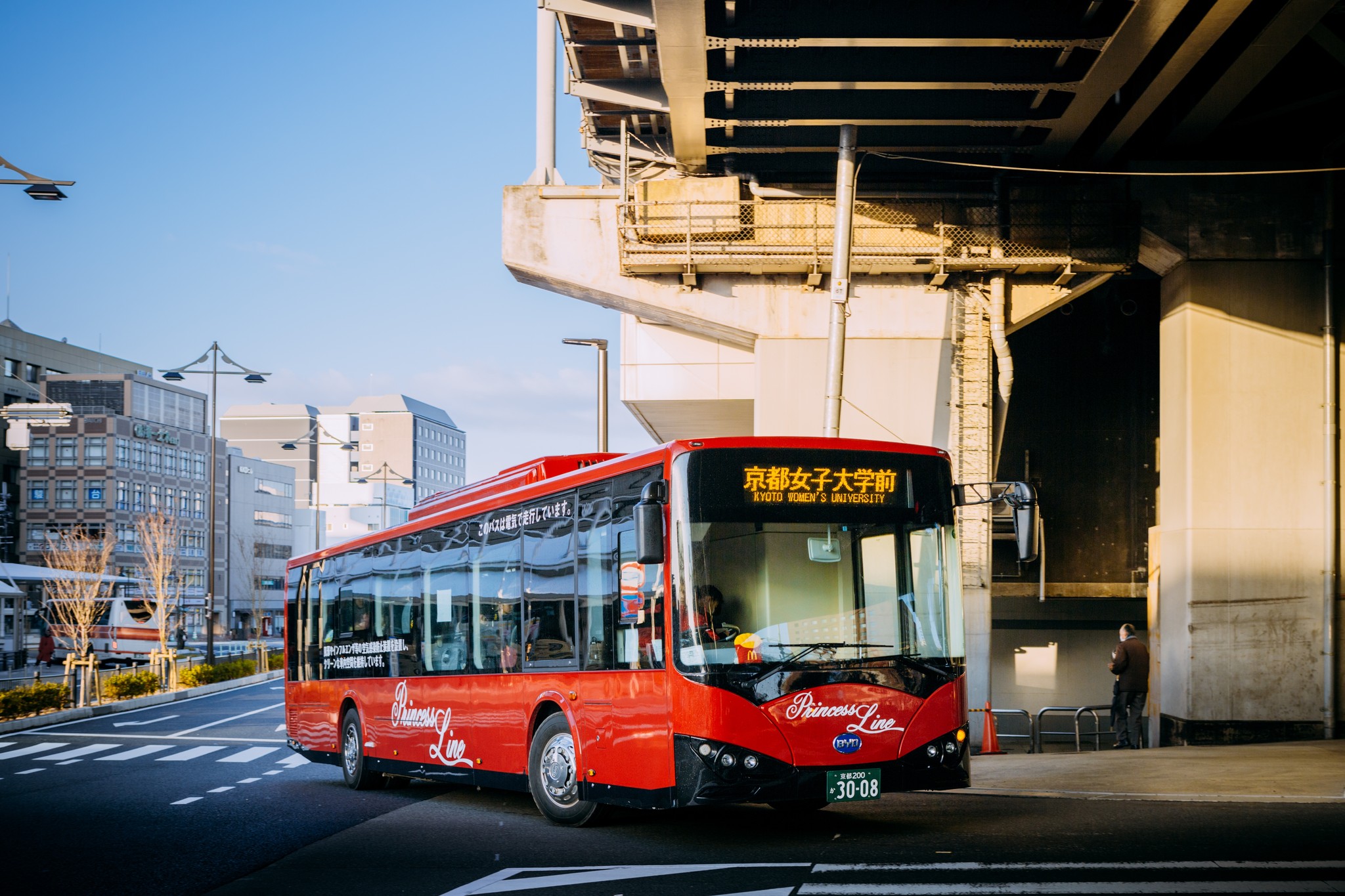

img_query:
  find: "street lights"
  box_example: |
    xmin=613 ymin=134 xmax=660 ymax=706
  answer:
xmin=277 ymin=422 xmax=359 ymax=551
xmin=354 ymin=462 xmax=417 ymax=529
xmin=157 ymin=340 xmax=273 ymax=669
xmin=561 ymin=337 xmax=608 ymax=453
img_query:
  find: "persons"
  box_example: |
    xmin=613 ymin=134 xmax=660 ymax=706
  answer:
xmin=693 ymin=584 xmax=730 ymax=641
xmin=354 ymin=598 xmax=370 ymax=612
xmin=494 ymin=604 xmax=516 ymax=628
xmin=639 ymin=579 xmax=720 ymax=654
xmin=448 ymin=605 xmax=494 ymax=670
xmin=1108 ymin=623 xmax=1148 ymax=750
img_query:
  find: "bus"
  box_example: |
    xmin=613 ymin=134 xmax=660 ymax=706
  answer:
xmin=283 ymin=437 xmax=1038 ymax=827
xmin=32 ymin=597 xmax=161 ymax=670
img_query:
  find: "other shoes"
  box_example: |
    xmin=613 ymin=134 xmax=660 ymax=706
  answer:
xmin=1130 ymin=744 xmax=1140 ymax=750
xmin=1112 ymin=738 xmax=1128 ymax=749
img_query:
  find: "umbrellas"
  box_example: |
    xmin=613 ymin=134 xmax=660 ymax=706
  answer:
xmin=1109 ymin=675 xmax=1120 ymax=731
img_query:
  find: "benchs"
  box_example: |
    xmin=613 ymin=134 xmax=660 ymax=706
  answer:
xmin=432 ymin=631 xmax=497 ymax=670
